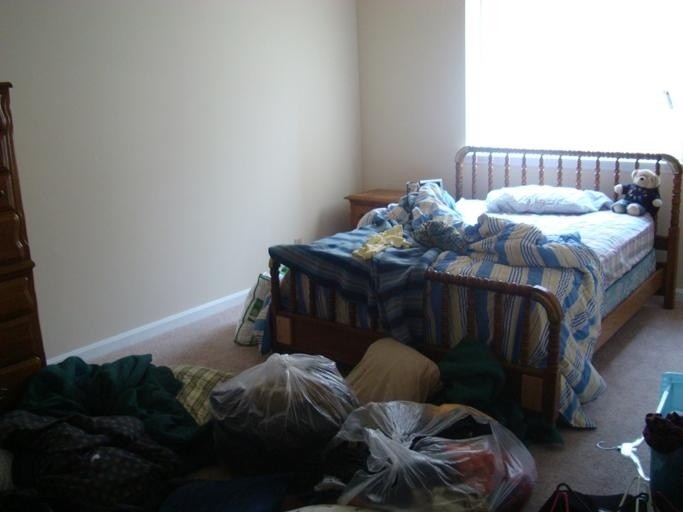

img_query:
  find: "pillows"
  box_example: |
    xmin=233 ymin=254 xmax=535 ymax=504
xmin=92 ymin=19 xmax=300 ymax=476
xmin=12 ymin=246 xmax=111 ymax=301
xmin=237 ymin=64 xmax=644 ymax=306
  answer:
xmin=484 ymin=184 xmax=614 ymax=216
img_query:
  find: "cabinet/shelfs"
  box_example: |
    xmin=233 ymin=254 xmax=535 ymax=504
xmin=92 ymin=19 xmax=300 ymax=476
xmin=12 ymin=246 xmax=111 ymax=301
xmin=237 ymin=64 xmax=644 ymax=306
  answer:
xmin=0 ymin=82 xmax=47 ymax=387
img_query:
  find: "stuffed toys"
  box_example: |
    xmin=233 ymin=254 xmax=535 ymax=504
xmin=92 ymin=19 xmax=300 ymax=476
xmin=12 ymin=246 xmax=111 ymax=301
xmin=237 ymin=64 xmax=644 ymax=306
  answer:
xmin=609 ymin=168 xmax=661 ymax=218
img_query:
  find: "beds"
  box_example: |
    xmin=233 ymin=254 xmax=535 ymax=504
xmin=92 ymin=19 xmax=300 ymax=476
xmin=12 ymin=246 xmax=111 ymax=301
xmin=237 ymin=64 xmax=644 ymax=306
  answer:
xmin=266 ymin=148 xmax=683 ymax=442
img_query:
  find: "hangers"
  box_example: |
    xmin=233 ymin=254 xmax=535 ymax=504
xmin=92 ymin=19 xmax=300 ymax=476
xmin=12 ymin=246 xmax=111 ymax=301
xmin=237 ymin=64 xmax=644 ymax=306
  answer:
xmin=547 ymin=430 xmax=650 ymax=511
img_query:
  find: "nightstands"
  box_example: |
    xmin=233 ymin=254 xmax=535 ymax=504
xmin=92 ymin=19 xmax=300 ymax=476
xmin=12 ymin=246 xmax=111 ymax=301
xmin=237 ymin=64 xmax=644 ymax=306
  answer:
xmin=347 ymin=188 xmax=409 ymax=228
